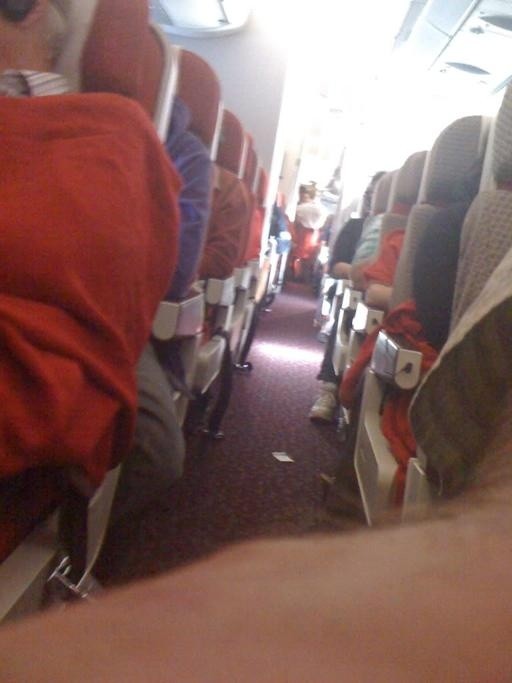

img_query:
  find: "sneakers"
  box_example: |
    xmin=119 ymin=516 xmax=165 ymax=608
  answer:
xmin=309 ymin=390 xmax=341 ymax=426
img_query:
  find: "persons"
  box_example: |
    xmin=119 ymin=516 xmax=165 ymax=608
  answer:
xmin=1 ymin=1 xmax=511 ymax=682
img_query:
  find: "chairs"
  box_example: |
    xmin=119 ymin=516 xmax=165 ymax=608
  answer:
xmin=317 ymin=78 xmax=512 ymax=527
xmin=1 ymin=0 xmax=269 ymax=621
